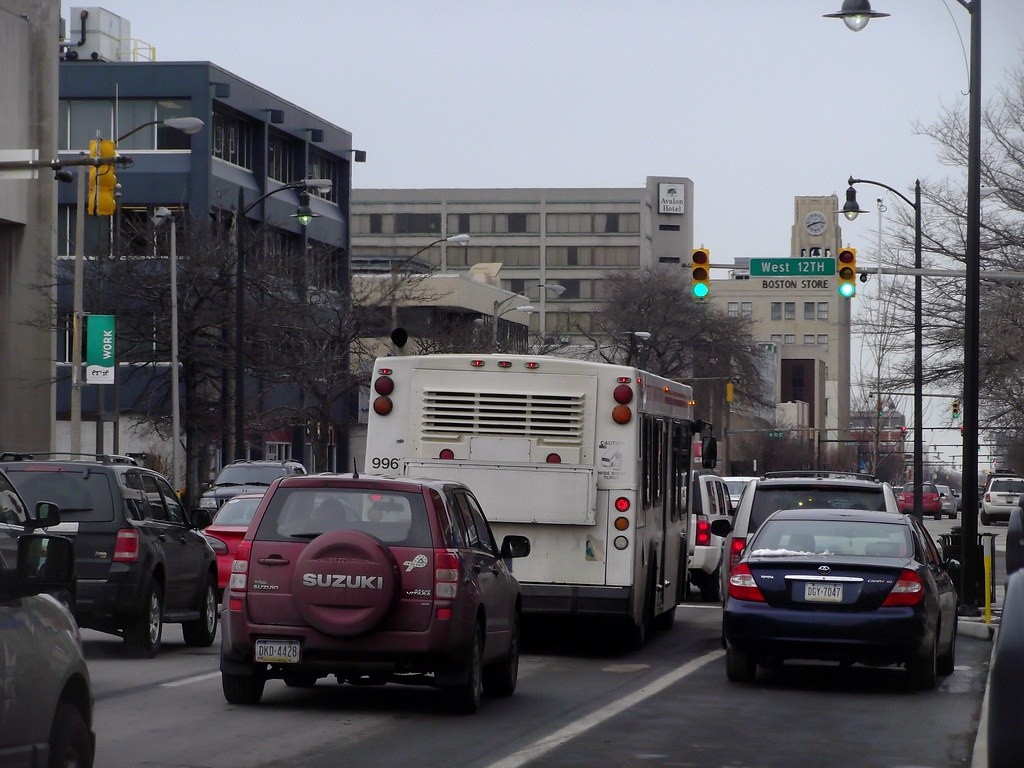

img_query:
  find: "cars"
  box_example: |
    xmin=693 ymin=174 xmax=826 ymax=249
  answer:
xmin=723 ymin=508 xmax=960 ymax=691
xmin=956 ymin=487 xmax=985 ymax=509
xmin=0 ymin=470 xmax=96 ymax=768
xmin=936 ymin=485 xmax=960 ymax=519
xmin=720 ymin=477 xmax=755 ymax=508
xmin=205 ymin=492 xmax=361 ymax=594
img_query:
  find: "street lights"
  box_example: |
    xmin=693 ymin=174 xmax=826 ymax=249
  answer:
xmin=493 ymin=284 xmax=567 ymax=341
xmin=72 ymin=115 xmax=205 ymax=463
xmin=234 ymin=176 xmax=332 ymax=458
xmin=596 ymin=332 xmax=652 ymax=362
xmin=833 ymin=175 xmax=924 ymax=522
xmin=390 ymin=235 xmax=471 ymax=350
xmin=825 ymin=1 xmax=983 ymax=616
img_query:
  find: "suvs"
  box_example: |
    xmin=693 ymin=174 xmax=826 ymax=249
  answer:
xmin=979 ymin=470 xmax=1024 ymax=525
xmin=197 ymin=458 xmax=305 ymax=516
xmin=710 ymin=470 xmax=901 ymax=618
xmin=0 ymin=452 xmax=219 ymax=659
xmin=220 ymin=469 xmax=531 ymax=714
xmin=689 ymin=470 xmax=733 ymax=602
xmin=898 ymin=481 xmax=944 ymax=520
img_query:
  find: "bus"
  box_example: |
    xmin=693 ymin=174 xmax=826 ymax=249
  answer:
xmin=364 ymin=355 xmax=718 ymax=646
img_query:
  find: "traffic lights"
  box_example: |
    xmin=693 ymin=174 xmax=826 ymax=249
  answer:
xmin=902 ymin=427 xmax=906 ymax=439
xmin=961 ymin=427 xmax=963 ymax=436
xmin=692 ymin=248 xmax=710 ymax=303
xmin=879 ymin=402 xmax=883 ymax=416
xmin=837 ymin=246 xmax=855 ymax=298
xmin=952 ymin=403 xmax=958 ymax=419
